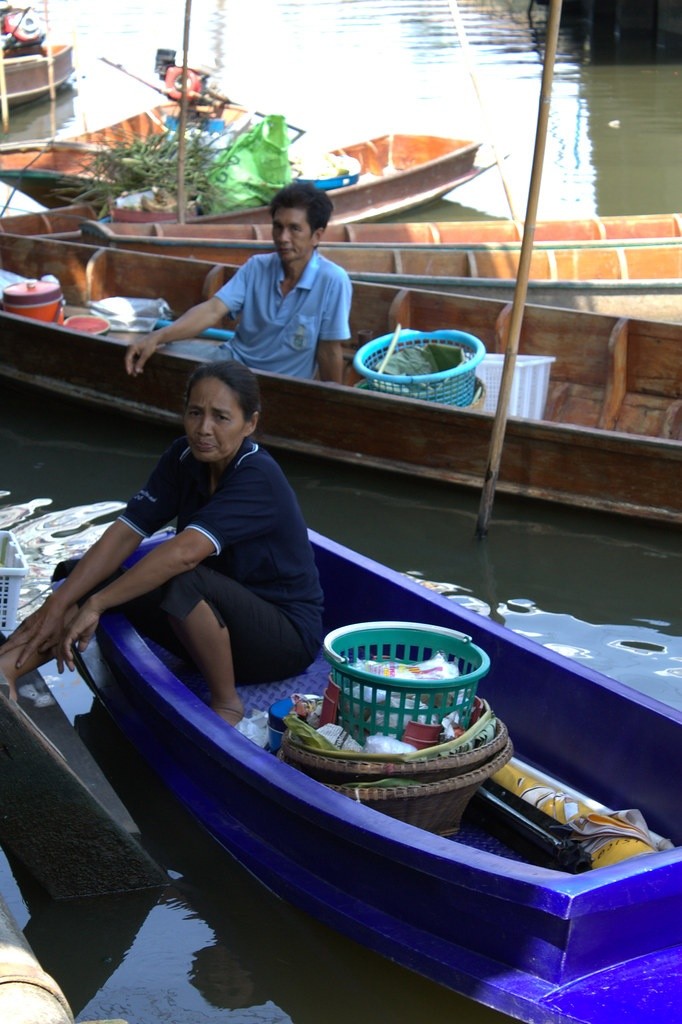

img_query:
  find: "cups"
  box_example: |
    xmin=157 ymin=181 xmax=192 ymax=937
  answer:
xmin=266 ymin=699 xmax=307 ymax=758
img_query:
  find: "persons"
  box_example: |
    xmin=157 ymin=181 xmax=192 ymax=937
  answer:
xmin=1 ymin=362 xmax=326 ymax=728
xmin=125 ymin=181 xmax=353 ymax=387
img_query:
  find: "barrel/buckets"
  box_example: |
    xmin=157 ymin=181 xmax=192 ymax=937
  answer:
xmin=2 ymin=282 xmax=67 ymax=328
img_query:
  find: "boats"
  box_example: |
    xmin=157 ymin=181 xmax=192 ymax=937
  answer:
xmin=49 ymin=527 xmax=682 ymax=1024
xmin=1 ymin=0 xmax=76 ymax=110
xmin=79 ymin=212 xmax=682 ymax=324
xmin=1 ymin=131 xmax=509 ymax=238
xmin=0 ymin=46 xmax=257 ymax=209
xmin=0 ymin=232 xmax=682 ymax=526
